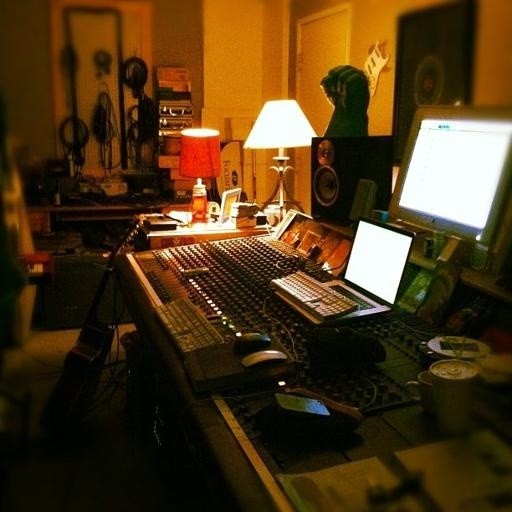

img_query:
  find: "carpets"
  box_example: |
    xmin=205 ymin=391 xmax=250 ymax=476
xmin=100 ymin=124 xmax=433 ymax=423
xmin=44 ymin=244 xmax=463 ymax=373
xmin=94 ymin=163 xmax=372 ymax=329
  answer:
xmin=28 ymin=255 xmax=135 ymax=331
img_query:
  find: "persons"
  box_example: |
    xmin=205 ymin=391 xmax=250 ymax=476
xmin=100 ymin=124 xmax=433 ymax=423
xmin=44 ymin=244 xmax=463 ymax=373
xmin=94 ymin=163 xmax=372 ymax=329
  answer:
xmin=321 ymin=64 xmax=370 ymax=138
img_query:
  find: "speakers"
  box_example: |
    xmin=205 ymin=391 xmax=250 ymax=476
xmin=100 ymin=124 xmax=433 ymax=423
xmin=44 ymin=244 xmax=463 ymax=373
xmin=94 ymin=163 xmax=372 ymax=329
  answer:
xmin=311 ymin=134 xmax=394 ymax=221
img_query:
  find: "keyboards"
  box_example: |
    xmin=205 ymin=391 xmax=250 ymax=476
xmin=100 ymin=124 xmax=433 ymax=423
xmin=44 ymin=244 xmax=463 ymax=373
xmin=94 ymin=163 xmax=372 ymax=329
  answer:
xmin=271 ymin=270 xmax=360 ymax=321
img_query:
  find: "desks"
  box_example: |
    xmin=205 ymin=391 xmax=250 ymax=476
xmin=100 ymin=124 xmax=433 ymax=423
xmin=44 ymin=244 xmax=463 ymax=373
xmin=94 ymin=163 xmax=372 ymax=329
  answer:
xmin=132 ymin=214 xmax=263 ymax=246
xmin=113 ymin=244 xmax=512 ymax=512
xmin=28 ymin=203 xmax=167 ymax=234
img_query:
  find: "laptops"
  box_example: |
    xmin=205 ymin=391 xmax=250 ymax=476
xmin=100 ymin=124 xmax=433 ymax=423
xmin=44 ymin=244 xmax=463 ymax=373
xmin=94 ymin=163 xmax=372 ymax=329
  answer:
xmin=274 ymin=219 xmax=416 ymax=325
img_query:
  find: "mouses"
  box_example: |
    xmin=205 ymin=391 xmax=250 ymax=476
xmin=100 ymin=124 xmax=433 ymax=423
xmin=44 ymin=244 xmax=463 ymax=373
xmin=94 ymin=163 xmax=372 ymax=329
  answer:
xmin=233 ymin=331 xmax=271 ymax=357
xmin=242 ymin=349 xmax=287 ymax=368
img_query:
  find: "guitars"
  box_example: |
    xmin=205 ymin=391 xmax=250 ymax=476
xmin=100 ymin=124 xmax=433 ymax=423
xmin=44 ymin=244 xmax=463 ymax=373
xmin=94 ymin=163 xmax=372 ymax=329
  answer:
xmin=37 ymin=215 xmax=151 ymax=462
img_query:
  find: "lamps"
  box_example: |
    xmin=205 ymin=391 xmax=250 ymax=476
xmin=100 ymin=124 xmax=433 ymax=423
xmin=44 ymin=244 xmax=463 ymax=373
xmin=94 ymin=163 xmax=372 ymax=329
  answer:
xmin=244 ymin=99 xmax=317 ymax=223
xmin=181 ymin=128 xmax=222 ymax=223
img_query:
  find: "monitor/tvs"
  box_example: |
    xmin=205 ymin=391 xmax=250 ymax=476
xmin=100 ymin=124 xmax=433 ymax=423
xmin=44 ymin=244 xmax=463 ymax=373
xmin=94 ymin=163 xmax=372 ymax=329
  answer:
xmin=388 ymin=107 xmax=512 ymax=262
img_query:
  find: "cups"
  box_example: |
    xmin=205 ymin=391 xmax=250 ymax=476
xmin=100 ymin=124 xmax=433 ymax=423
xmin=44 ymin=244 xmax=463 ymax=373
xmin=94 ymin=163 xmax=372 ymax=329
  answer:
xmin=404 ymin=360 xmax=479 ymax=434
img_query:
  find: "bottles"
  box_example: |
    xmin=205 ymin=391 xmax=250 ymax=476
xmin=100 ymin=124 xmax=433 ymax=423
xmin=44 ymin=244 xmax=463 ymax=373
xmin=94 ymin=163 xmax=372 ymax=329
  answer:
xmin=190 ymin=185 xmax=208 ymax=224
